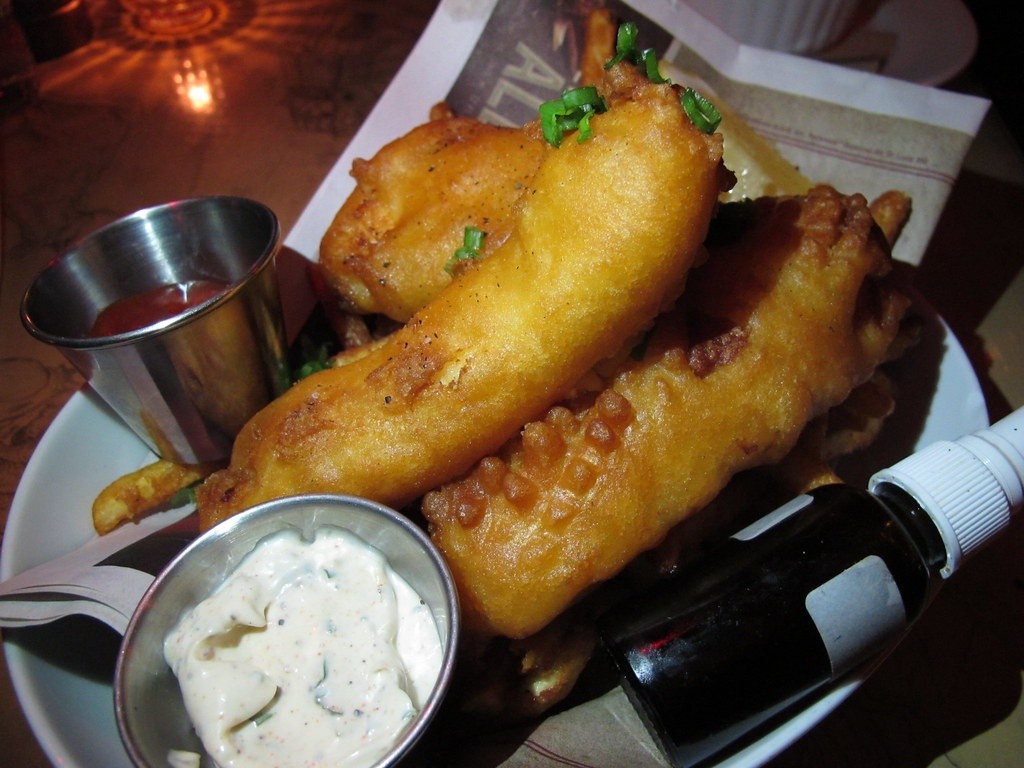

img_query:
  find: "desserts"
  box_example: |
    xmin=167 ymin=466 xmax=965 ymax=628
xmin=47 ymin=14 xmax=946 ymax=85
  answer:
xmin=163 ymin=529 xmax=447 ymax=767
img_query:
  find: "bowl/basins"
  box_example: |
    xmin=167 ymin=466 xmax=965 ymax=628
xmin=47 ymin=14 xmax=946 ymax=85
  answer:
xmin=111 ymin=492 xmax=460 ymax=767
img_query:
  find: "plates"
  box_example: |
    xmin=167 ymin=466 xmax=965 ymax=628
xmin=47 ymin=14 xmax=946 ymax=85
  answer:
xmin=0 ymin=304 xmax=997 ymax=767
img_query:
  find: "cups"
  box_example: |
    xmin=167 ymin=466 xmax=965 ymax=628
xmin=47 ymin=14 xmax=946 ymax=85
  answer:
xmin=21 ymin=193 xmax=282 ymax=468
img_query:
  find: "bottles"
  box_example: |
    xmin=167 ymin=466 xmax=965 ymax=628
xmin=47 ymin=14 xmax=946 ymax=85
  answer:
xmin=588 ymin=407 xmax=1024 ymax=768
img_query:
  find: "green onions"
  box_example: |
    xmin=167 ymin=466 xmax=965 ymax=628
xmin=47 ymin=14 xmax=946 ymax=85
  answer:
xmin=293 ymin=22 xmax=722 ymax=384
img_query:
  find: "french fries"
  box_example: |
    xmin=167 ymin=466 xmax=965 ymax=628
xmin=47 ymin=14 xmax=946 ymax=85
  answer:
xmin=90 ymin=456 xmax=216 ymax=535
